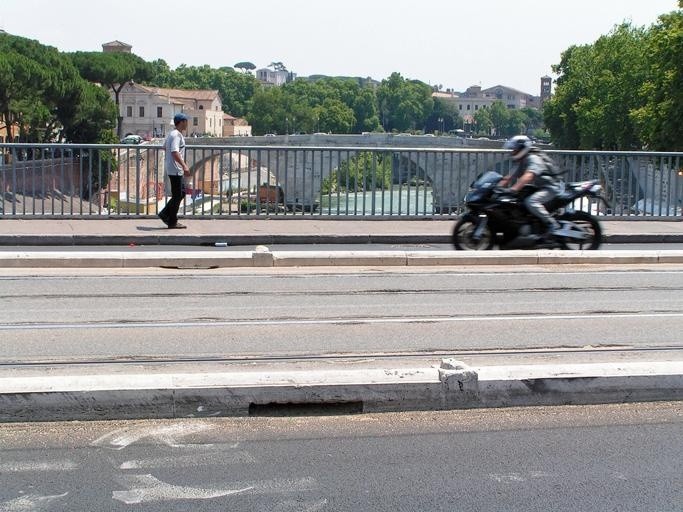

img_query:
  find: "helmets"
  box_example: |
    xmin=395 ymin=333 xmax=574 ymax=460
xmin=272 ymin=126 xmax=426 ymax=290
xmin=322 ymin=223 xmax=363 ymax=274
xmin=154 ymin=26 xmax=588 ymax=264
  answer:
xmin=502 ymin=134 xmax=532 ymax=162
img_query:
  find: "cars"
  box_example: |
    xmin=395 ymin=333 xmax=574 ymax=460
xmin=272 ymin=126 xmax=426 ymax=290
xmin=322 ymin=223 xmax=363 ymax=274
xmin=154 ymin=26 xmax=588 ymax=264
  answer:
xmin=263 ymin=134 xmax=275 ymax=136
xmin=119 ymin=134 xmax=142 ymax=144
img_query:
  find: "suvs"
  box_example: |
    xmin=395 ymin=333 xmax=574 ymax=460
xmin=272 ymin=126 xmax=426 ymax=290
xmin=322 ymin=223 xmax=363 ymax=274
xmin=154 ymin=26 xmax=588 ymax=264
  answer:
xmin=289 ymin=131 xmax=307 ymax=136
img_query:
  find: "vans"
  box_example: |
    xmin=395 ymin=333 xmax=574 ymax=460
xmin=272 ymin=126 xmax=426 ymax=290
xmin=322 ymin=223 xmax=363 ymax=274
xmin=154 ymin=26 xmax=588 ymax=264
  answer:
xmin=313 ymin=132 xmax=326 ymax=136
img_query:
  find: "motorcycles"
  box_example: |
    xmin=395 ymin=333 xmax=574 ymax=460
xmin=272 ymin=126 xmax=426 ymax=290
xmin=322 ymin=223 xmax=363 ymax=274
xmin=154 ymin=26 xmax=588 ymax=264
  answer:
xmin=450 ymin=169 xmax=612 ymax=250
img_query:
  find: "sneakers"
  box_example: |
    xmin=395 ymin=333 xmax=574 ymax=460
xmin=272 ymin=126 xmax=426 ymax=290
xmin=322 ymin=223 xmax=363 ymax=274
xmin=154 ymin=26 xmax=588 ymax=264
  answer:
xmin=157 ymin=211 xmax=187 ymax=229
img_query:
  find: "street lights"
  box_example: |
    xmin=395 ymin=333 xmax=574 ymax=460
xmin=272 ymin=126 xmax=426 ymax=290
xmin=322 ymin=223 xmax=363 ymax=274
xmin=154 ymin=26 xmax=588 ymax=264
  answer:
xmin=285 ymin=117 xmax=289 ymax=135
xmin=292 ymin=117 xmax=295 ymax=134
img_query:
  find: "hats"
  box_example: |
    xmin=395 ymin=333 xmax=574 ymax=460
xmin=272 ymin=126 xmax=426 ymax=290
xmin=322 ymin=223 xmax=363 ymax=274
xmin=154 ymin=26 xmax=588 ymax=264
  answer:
xmin=172 ymin=113 xmax=193 ymax=127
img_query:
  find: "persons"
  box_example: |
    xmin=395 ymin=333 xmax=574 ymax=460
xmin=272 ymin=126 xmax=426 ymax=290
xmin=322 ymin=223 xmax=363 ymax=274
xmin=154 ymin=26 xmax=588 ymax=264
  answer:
xmin=156 ymin=112 xmax=191 ymax=231
xmin=493 ymin=134 xmax=566 ymax=239
